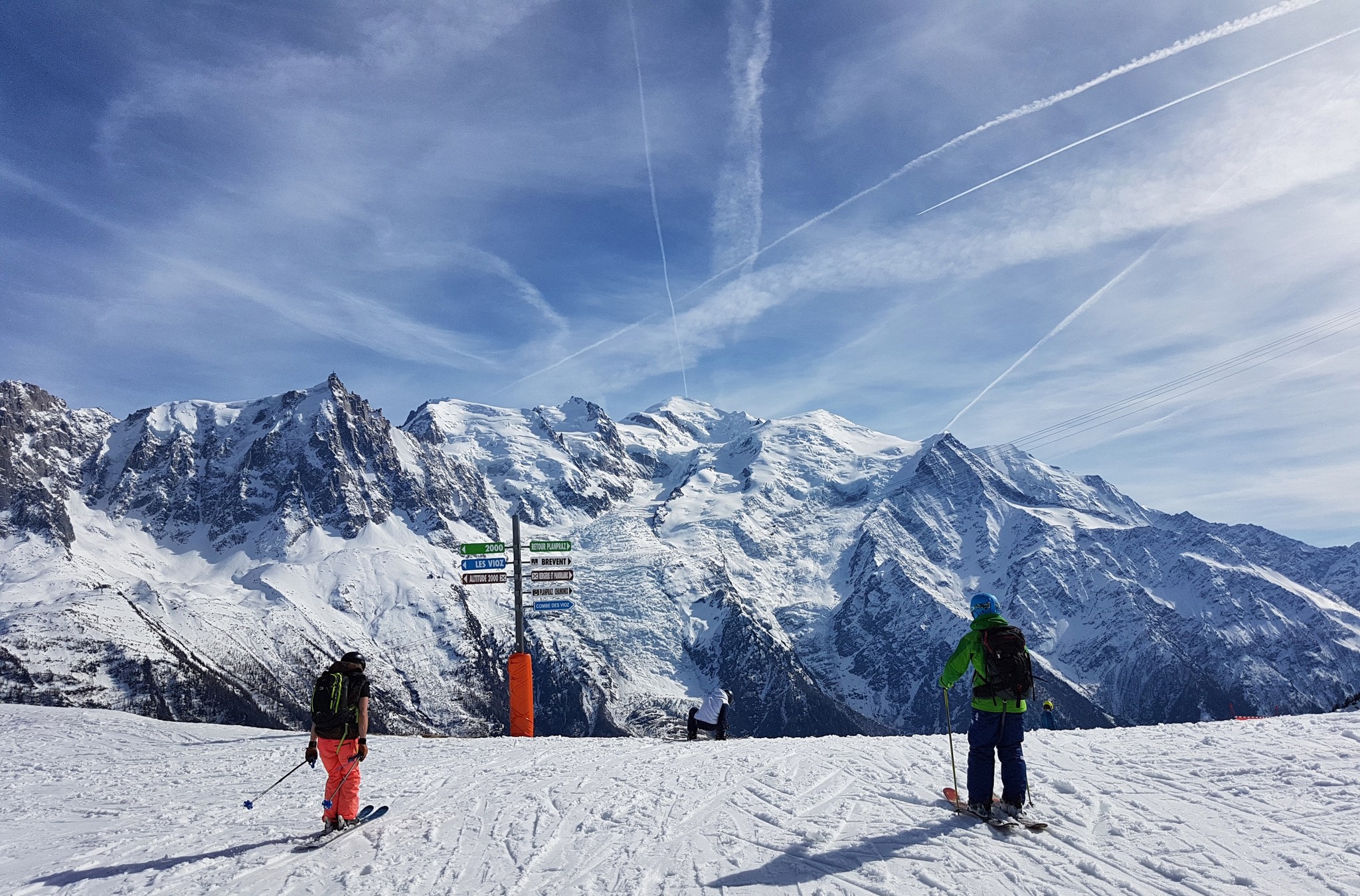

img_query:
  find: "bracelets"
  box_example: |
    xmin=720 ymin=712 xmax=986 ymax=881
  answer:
xmin=358 ymin=738 xmax=366 ymax=744
xmin=308 ymin=741 xmax=317 ymax=747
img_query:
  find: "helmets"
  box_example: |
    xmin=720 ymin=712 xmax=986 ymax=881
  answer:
xmin=725 ymin=690 xmax=734 ymax=702
xmin=1043 ymin=701 xmax=1053 ymax=711
xmin=343 ymin=651 xmax=366 ymax=671
xmin=971 ymin=593 xmax=1000 ymax=619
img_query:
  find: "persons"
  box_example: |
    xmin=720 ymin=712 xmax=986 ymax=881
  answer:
xmin=937 ymin=594 xmax=1028 ymax=817
xmin=687 ymin=688 xmax=734 ymax=740
xmin=1041 ymin=701 xmax=1058 ymax=730
xmin=305 ymin=651 xmax=370 ymax=833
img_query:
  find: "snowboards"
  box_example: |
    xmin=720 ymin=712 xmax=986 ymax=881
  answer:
xmin=661 ymin=733 xmax=750 ymax=742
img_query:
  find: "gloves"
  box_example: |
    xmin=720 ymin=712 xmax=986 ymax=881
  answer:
xmin=357 ymin=743 xmax=368 ymax=762
xmin=718 ymin=732 xmax=726 ymax=741
xmin=305 ymin=747 xmax=318 ymax=763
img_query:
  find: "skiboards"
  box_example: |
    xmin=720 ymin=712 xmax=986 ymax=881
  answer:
xmin=943 ymin=788 xmax=1048 ymax=830
xmin=291 ymin=802 xmax=392 ymax=849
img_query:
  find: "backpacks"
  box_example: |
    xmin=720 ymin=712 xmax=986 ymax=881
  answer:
xmin=976 ymin=624 xmax=1033 ymax=700
xmin=311 ymin=670 xmax=369 ymax=728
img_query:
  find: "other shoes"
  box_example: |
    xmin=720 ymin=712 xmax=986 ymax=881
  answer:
xmin=687 ymin=730 xmax=697 ymax=740
xmin=344 ymin=818 xmax=359 ymax=826
xmin=716 ymin=733 xmax=729 ymax=740
xmin=1000 ymin=801 xmax=1024 ymax=819
xmin=330 ymin=822 xmax=339 ymax=830
xmin=968 ymin=803 xmax=992 ymax=817
